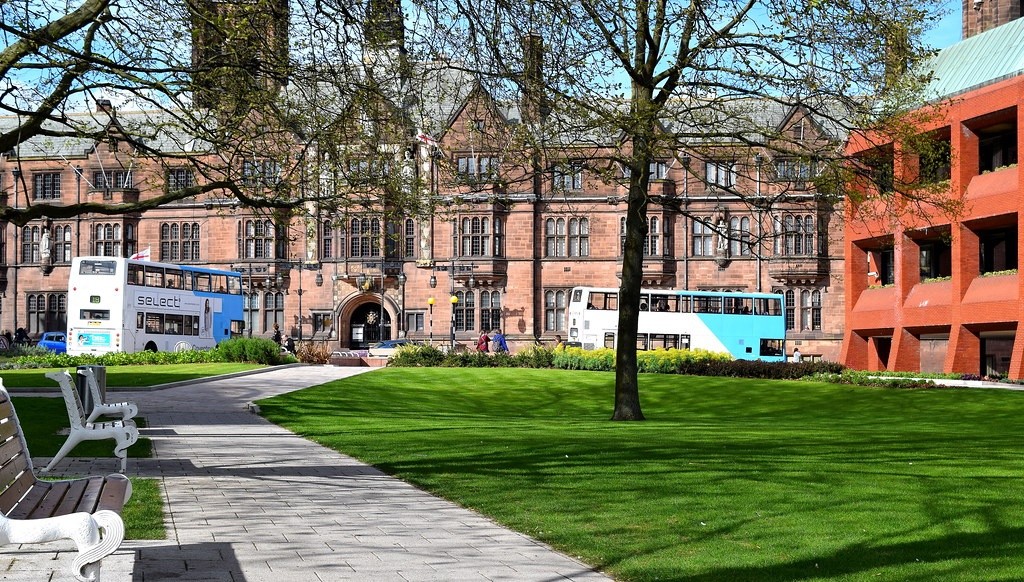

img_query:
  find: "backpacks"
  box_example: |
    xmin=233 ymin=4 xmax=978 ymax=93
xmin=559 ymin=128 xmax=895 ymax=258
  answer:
xmin=492 ymin=340 xmax=500 ymax=352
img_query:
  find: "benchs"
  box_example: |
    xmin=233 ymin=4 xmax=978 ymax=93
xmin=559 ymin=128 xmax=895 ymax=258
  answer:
xmin=77 ymin=369 xmax=138 ymax=423
xmin=0 ymin=376 xmax=133 ymax=582
xmin=40 ymin=370 xmax=139 ymax=473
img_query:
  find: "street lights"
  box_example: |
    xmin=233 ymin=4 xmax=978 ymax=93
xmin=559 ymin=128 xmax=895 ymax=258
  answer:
xmin=359 ymin=257 xmax=407 ymax=341
xmin=429 ymin=260 xmax=475 ymax=352
xmin=229 ymin=263 xmax=272 ymax=339
xmin=450 ymin=294 xmax=459 ymax=348
xmin=275 ymin=257 xmax=323 ymax=343
xmin=428 ymin=298 xmax=435 ymax=347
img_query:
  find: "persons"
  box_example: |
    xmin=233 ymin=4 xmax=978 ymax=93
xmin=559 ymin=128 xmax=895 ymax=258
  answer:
xmin=271 ymin=323 xmax=280 ymax=346
xmin=761 ymin=340 xmax=773 ymax=351
xmin=403 ymin=151 xmax=415 ymax=194
xmin=646 ymin=301 xmax=670 ymax=311
xmin=794 ymin=348 xmax=800 ymax=362
xmin=168 ymin=279 xmax=173 ymax=288
xmin=493 ymin=329 xmax=509 ymax=352
xmin=219 ymin=286 xmax=225 ymax=293
xmin=42 ymin=229 xmax=50 ymax=251
xmin=15 ymin=327 xmax=30 ymax=342
xmin=554 ymin=336 xmax=561 ymax=344
xmin=0 ymin=330 xmax=11 ymax=342
xmin=716 ymin=220 xmax=726 ymax=249
xmin=284 ymin=335 xmax=294 ymax=351
xmin=476 ymin=329 xmax=491 ymax=353
xmin=744 ymin=306 xmax=757 ymax=314
xmin=535 ymin=334 xmax=542 ymax=344
xmin=204 ymin=299 xmax=212 ymax=331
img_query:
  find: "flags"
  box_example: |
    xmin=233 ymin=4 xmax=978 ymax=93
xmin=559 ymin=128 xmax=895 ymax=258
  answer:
xmin=131 ymin=248 xmax=150 ymax=261
xmin=417 ymin=130 xmax=436 ymax=146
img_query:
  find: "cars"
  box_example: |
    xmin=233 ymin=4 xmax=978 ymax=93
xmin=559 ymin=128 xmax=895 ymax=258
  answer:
xmin=373 ymin=339 xmax=425 ymax=348
xmin=38 ymin=332 xmax=66 ymax=355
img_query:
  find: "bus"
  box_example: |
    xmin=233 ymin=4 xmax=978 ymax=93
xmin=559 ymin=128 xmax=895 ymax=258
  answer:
xmin=566 ymin=285 xmax=786 ymax=362
xmin=67 ymin=256 xmax=244 ymax=358
